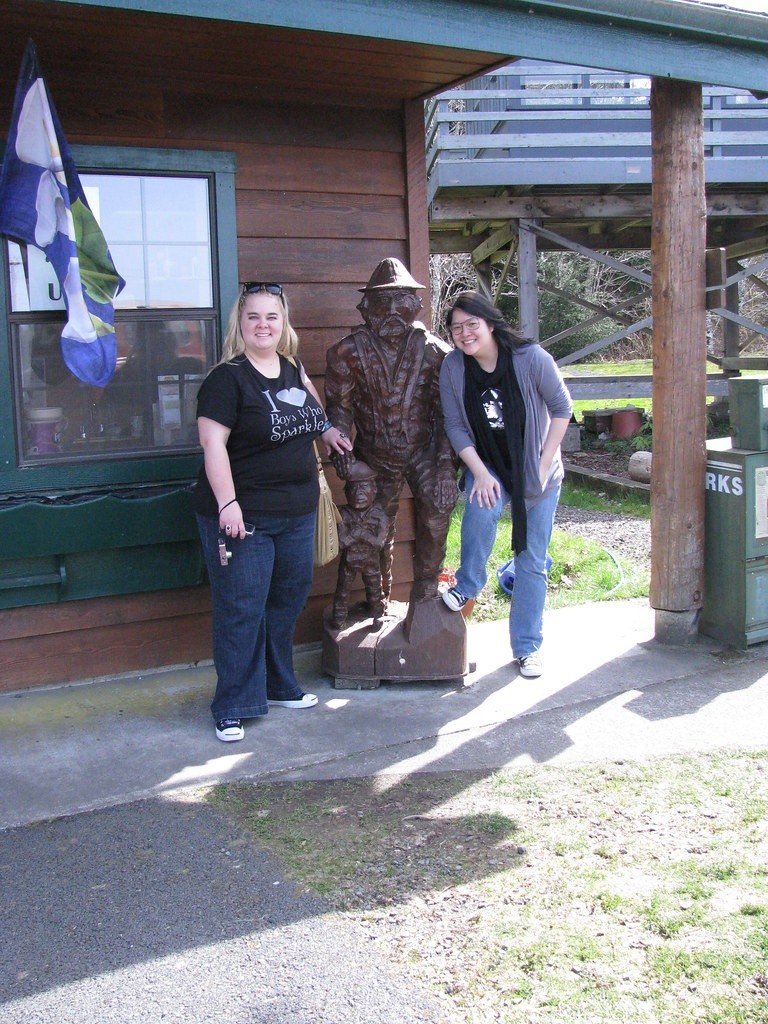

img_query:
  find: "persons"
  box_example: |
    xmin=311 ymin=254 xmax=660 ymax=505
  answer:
xmin=323 ymin=257 xmax=459 ymax=610
xmin=190 ymin=279 xmax=354 ymax=741
xmin=331 ymin=461 xmax=390 ymax=630
xmin=441 ymin=290 xmax=572 ymax=678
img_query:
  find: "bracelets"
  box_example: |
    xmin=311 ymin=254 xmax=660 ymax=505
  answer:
xmin=320 ymin=420 xmax=332 ymax=435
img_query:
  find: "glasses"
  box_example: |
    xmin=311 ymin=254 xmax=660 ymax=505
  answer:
xmin=242 ymin=281 xmax=285 ymax=307
xmin=447 ymin=317 xmax=480 ymax=335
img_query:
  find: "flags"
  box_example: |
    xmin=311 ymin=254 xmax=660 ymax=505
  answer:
xmin=0 ymin=43 xmax=125 ymax=388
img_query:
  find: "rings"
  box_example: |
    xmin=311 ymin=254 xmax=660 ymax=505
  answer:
xmin=226 ymin=525 xmax=231 ymax=531
xmin=340 ymin=433 xmax=346 ymax=437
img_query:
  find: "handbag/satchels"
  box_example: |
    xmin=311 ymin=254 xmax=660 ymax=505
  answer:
xmin=314 ymin=471 xmax=344 ymax=567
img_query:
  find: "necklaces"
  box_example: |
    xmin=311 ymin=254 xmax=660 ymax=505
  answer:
xmin=246 ymin=346 xmax=279 ymax=377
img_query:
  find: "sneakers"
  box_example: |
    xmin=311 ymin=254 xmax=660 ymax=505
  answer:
xmin=216 ymin=718 xmax=245 ymax=741
xmin=442 ymin=587 xmax=469 ymax=612
xmin=266 ymin=693 xmax=318 ymax=709
xmin=518 ymin=656 xmax=541 ymax=677
